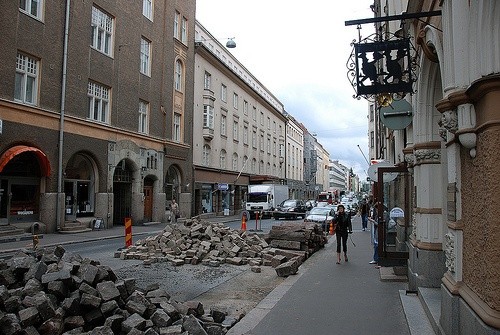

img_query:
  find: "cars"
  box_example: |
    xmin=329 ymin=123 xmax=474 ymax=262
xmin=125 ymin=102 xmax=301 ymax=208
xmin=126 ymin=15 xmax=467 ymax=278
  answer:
xmin=304 ymin=207 xmax=338 ymax=231
xmin=303 ymin=189 xmax=366 ymax=217
xmin=272 ymin=198 xmax=307 ymax=220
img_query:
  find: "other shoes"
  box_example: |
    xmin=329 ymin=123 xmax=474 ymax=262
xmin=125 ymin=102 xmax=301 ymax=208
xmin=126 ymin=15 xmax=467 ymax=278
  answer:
xmin=362 ymin=228 xmax=365 ymax=231
xmin=345 ymin=257 xmax=348 ymax=262
xmin=369 ymin=260 xmax=377 ymax=264
xmin=337 ymin=258 xmax=341 ymax=264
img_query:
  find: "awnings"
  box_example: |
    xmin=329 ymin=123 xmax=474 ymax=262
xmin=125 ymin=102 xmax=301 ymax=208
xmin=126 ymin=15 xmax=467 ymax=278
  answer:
xmin=0 ymin=145 xmax=51 ymax=177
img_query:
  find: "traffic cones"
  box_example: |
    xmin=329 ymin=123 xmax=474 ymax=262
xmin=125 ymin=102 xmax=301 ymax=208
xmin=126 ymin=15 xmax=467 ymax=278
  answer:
xmin=240 ymin=216 xmax=247 ymax=231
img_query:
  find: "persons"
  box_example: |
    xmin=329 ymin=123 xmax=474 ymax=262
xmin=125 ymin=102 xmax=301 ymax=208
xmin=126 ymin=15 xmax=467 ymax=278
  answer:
xmin=368 ymin=202 xmax=387 ymax=264
xmin=332 ymin=205 xmax=352 ymax=264
xmin=170 ymin=199 xmax=178 ymax=224
xmin=360 ymin=198 xmax=378 ymax=231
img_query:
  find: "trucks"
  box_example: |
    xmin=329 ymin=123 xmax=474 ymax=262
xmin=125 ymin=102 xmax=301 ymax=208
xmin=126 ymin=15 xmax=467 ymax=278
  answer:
xmin=246 ymin=183 xmax=289 ymax=218
